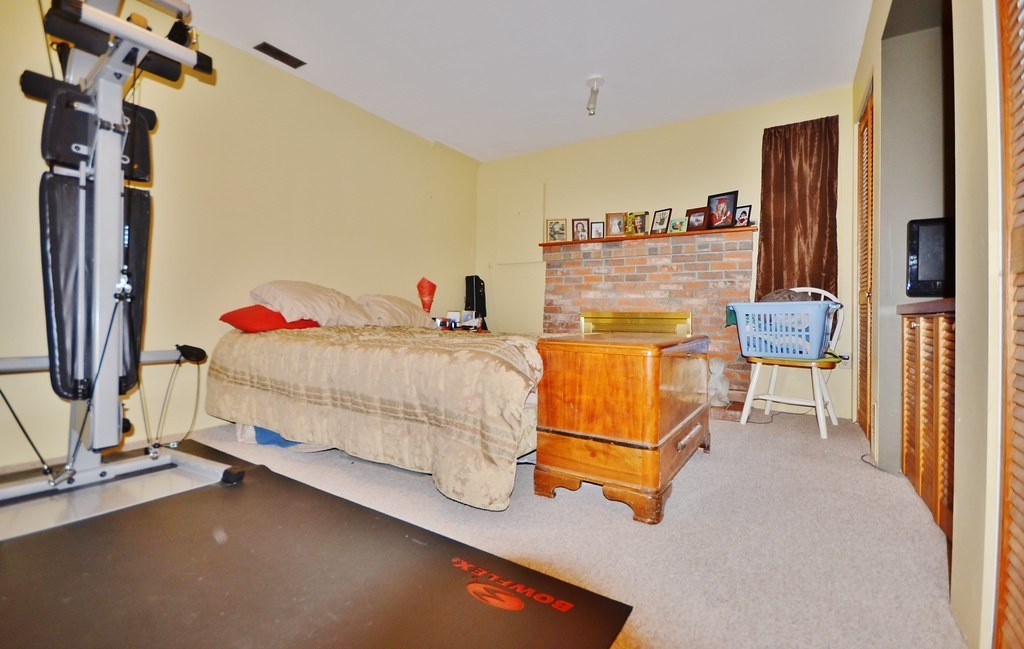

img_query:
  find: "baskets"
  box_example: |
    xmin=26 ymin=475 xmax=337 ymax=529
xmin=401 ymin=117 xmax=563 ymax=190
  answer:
xmin=727 ymin=301 xmax=841 ymax=358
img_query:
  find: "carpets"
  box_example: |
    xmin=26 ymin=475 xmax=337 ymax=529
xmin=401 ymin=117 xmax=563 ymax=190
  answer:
xmin=0 ymin=437 xmax=634 ymax=649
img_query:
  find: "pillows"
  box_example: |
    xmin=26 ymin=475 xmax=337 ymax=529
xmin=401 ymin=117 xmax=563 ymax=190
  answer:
xmin=250 ymin=279 xmax=377 ymax=327
xmin=355 ymin=294 xmax=439 ymax=329
xmin=219 ymin=304 xmax=320 ymax=333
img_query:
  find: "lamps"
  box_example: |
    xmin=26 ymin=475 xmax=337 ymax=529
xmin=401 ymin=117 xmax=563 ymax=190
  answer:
xmin=584 ymin=75 xmax=605 ymax=116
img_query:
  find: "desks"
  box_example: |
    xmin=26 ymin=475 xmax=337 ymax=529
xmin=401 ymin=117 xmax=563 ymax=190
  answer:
xmin=534 ymin=332 xmax=712 ymax=525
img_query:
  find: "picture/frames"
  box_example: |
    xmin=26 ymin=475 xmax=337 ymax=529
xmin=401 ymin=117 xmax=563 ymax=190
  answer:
xmin=731 ymin=204 xmax=752 ymax=228
xmin=591 ymin=222 xmax=604 ymax=239
xmin=546 ymin=218 xmax=567 ymax=242
xmin=705 ymin=190 xmax=739 ymax=230
xmin=628 ymin=211 xmax=650 ymax=236
xmin=685 ymin=206 xmax=710 ymax=231
xmin=572 ymin=218 xmax=589 ymax=241
xmin=606 ymin=212 xmax=628 ymax=238
xmin=650 ymin=208 xmax=672 ymax=235
xmin=461 ymin=310 xmax=475 ymax=329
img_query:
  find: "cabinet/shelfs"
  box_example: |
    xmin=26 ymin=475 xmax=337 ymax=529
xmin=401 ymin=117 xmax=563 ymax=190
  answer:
xmin=859 ymin=0 xmax=1024 ymax=649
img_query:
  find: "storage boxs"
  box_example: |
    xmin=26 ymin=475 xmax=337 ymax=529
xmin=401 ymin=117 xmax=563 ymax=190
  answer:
xmin=727 ymin=301 xmax=841 ymax=360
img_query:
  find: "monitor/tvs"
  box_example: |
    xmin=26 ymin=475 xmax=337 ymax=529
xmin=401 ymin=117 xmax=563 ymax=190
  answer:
xmin=905 ymin=216 xmax=946 ymax=299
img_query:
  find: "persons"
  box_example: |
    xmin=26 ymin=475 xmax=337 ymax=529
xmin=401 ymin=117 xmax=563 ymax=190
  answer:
xmin=576 ymin=223 xmax=586 ymax=233
xmin=738 ymin=211 xmax=747 ymax=225
xmin=635 ymin=216 xmax=644 ymax=233
xmin=711 ymin=198 xmax=732 ymax=225
xmin=549 ymin=222 xmax=560 ymax=239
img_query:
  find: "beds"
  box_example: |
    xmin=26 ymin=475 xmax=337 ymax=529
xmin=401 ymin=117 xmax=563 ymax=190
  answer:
xmin=204 ymin=279 xmax=545 ymax=513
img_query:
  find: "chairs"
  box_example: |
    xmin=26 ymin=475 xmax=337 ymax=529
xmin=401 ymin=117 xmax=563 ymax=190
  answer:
xmin=739 ymin=287 xmax=845 ymax=439
